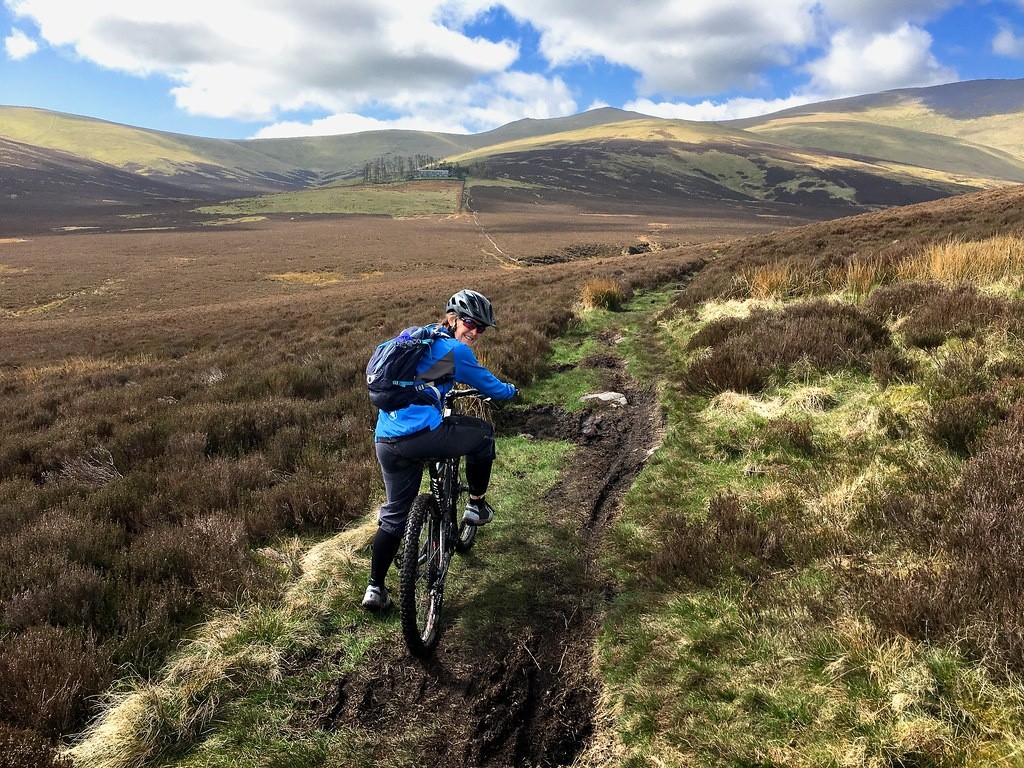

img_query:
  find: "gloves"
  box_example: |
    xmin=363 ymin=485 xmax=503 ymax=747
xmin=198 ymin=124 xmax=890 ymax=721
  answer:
xmin=508 ymin=383 xmax=526 ymax=406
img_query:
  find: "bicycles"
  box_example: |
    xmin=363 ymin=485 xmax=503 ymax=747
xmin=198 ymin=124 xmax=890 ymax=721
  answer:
xmin=395 ymin=383 xmax=519 ymax=657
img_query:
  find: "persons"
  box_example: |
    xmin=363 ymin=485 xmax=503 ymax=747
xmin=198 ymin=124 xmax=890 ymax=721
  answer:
xmin=361 ymin=289 xmax=515 ymax=609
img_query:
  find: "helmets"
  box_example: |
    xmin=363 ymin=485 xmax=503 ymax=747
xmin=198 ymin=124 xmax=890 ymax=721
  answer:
xmin=445 ymin=289 xmax=497 ymax=327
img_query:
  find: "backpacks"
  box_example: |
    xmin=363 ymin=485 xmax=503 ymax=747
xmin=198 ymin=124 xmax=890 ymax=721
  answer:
xmin=365 ymin=324 xmax=453 ymax=412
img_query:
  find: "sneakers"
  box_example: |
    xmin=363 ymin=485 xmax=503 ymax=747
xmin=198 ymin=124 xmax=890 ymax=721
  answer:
xmin=361 ymin=579 xmax=393 ymax=611
xmin=463 ymin=501 xmax=496 ymax=524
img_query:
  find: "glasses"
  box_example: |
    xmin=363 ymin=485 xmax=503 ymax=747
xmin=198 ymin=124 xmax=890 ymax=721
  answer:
xmin=459 ymin=315 xmax=487 ymax=333
